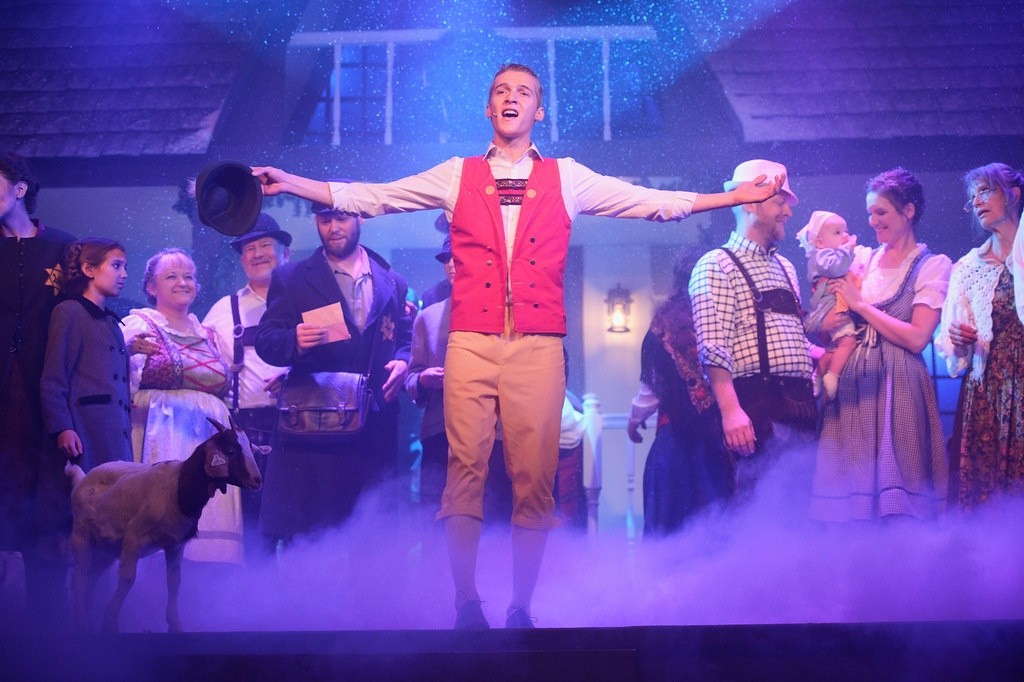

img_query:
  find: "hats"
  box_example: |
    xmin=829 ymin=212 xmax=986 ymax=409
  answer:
xmin=197 ymin=159 xmax=262 ymax=236
xmin=229 ymin=212 xmax=292 ymax=254
xmin=723 ymin=159 xmax=798 ymax=207
xmin=807 ymin=211 xmax=836 ymax=244
xmin=311 ymin=179 xmax=359 ymax=216
xmin=435 ymin=234 xmax=452 ymax=263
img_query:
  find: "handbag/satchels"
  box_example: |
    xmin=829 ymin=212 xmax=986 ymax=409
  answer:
xmin=769 ymin=390 xmax=818 ymax=424
xmin=277 ymin=370 xmax=374 ymax=437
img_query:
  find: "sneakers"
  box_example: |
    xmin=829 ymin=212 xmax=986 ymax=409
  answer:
xmin=452 ymin=590 xmax=489 ymax=630
xmin=505 ymin=606 xmax=538 ymax=628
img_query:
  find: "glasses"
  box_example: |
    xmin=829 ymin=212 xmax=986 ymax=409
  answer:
xmin=963 ymin=188 xmax=1002 ymax=213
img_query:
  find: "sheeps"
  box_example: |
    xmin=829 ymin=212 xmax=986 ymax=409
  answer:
xmin=66 ymin=415 xmax=273 ymax=637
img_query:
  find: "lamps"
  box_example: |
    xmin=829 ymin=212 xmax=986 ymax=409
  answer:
xmin=602 ymin=282 xmax=635 ymax=334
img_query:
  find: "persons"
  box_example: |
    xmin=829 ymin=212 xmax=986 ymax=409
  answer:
xmin=624 ymin=245 xmax=737 ymax=544
xmin=42 ymin=236 xmax=134 ymax=596
xmin=686 ymin=157 xmax=826 ymax=511
xmin=799 ymin=165 xmax=956 ymax=527
xmin=932 ymin=163 xmax=1024 ymax=510
xmin=1 ymin=150 xmax=83 ymax=626
xmin=248 ymin=65 xmax=790 ymax=629
xmin=252 ymin=177 xmax=419 ymax=536
xmin=403 ymin=212 xmax=589 ymax=529
xmin=795 ymin=207 xmax=863 ymax=401
xmin=203 ymin=210 xmax=292 ymax=534
xmin=119 ymin=247 xmax=244 ymax=562
